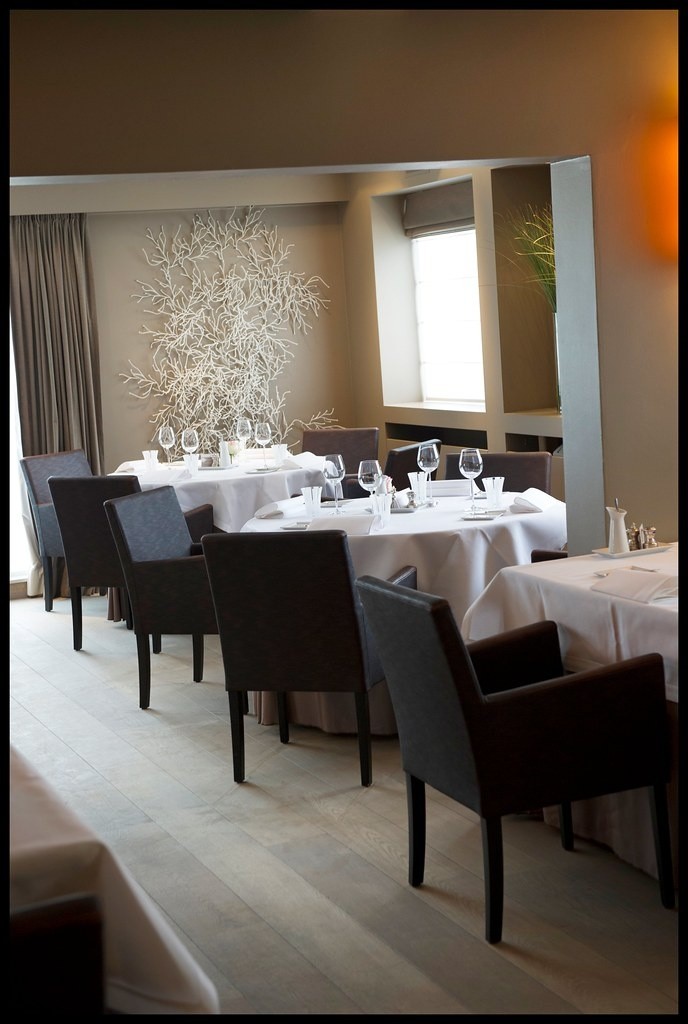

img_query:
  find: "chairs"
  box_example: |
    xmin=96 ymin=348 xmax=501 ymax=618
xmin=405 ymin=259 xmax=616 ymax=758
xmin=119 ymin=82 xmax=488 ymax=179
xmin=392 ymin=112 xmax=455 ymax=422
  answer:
xmin=301 ymin=428 xmax=381 ymax=498
xmin=292 ymin=439 xmax=442 ymax=502
xmin=46 ymin=475 xmax=215 ymax=652
xmin=19 ymin=449 xmax=108 ymax=613
xmin=444 ymin=452 xmax=552 ymax=494
xmin=353 ymin=574 xmax=676 ymax=946
xmin=200 ymin=531 xmax=419 ymax=789
xmin=103 ymin=484 xmax=219 ymax=709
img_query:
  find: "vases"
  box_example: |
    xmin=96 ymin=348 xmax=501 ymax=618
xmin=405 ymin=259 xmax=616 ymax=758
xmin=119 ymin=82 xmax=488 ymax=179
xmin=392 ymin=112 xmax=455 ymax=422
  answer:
xmin=552 ymin=312 xmax=563 ymax=415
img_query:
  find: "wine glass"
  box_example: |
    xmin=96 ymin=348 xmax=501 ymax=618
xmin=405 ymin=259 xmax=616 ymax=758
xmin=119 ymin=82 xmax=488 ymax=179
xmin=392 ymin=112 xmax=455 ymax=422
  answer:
xmin=359 ymin=461 xmax=382 ymax=513
xmin=159 ymin=427 xmax=175 ymax=463
xmin=322 ymin=454 xmax=346 ymax=516
xmin=255 ymin=423 xmax=271 ymax=467
xmin=459 ymin=449 xmax=483 ymax=501
xmin=237 ymin=420 xmax=251 ymax=450
xmin=418 ymin=444 xmax=440 ymax=503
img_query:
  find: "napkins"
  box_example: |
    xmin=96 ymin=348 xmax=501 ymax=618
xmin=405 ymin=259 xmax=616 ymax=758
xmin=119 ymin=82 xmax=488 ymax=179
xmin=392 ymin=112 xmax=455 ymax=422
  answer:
xmin=163 ymin=464 xmax=198 ymax=483
xmin=427 ymin=479 xmax=480 ymax=498
xmin=254 ymin=495 xmax=306 ymax=519
xmin=509 ymin=486 xmax=556 ymax=514
xmin=590 ymin=569 xmax=678 ymax=604
xmin=281 ymin=451 xmax=320 ymax=470
xmin=114 ymin=460 xmax=159 ymax=476
xmin=306 ymin=513 xmax=384 ymax=536
xmin=246 ymin=448 xmax=291 ymax=459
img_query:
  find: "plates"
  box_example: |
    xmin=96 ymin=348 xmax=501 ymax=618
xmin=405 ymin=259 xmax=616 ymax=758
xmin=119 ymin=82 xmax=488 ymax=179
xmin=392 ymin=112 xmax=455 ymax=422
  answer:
xmin=280 ymin=522 xmax=310 ymax=529
xmin=246 ymin=470 xmax=271 ymax=473
xmin=593 ymin=566 xmax=655 ymax=576
xmin=321 ymin=501 xmax=351 ymax=507
xmin=592 ymin=542 xmax=673 ymax=558
xmin=462 ymin=513 xmax=503 ymax=519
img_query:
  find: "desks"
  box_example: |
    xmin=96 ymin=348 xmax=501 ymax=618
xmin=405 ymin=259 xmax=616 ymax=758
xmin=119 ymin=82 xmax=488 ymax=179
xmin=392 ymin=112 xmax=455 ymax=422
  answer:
xmin=460 ymin=541 xmax=679 ymax=880
xmin=239 ymin=489 xmax=567 ymax=737
xmin=107 ymin=447 xmax=344 ymax=623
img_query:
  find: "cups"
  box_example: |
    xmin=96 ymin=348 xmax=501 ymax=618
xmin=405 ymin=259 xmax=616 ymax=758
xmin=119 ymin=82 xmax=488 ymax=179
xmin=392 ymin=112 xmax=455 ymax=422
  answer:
xmin=182 ymin=430 xmax=198 ymax=454
xmin=183 ymin=455 xmax=199 ymax=474
xmin=301 ymin=487 xmax=321 ymax=514
xmin=606 ymin=506 xmax=629 ymax=552
xmin=371 ymin=494 xmax=392 ymax=515
xmin=408 ymin=472 xmax=427 ymax=499
xmin=482 ymin=477 xmax=505 ymax=501
xmin=143 ymin=450 xmax=158 ymax=467
xmin=272 ymin=444 xmax=287 ymax=462
